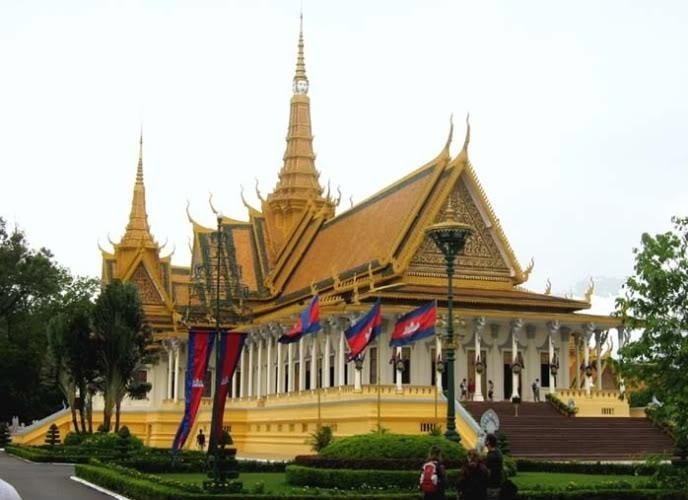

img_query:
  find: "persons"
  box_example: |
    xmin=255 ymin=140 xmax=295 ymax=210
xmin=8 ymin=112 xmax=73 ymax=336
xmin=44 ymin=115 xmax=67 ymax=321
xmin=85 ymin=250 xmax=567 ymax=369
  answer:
xmin=460 ymin=378 xmax=468 ymax=401
xmin=419 ymin=447 xmax=448 ymax=500
xmin=532 ymin=378 xmax=540 ymax=402
xmin=457 ymin=448 xmax=489 ymax=500
xmin=468 ymin=378 xmax=475 ymax=401
xmin=482 ymin=433 xmax=503 ymax=500
xmin=196 ymin=429 xmax=205 ymax=451
xmin=581 ymin=366 xmax=597 ymax=389
xmin=488 ymin=380 xmax=494 ymax=400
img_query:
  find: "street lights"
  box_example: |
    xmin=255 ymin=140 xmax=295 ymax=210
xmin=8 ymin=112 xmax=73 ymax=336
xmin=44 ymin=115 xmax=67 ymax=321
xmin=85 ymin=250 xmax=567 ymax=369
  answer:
xmin=422 ymin=195 xmax=474 ymax=444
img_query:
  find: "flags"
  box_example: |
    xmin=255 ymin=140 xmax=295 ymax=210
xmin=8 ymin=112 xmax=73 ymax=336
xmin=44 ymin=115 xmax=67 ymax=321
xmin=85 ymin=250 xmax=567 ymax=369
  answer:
xmin=344 ymin=298 xmax=384 ymax=361
xmin=389 ymin=298 xmax=436 ymax=347
xmin=277 ymin=295 xmax=322 ymax=344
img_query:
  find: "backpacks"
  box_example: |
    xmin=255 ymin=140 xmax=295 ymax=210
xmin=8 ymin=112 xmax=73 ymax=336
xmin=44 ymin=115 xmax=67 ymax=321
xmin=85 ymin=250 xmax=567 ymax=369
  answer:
xmin=418 ymin=460 xmax=444 ymax=493
